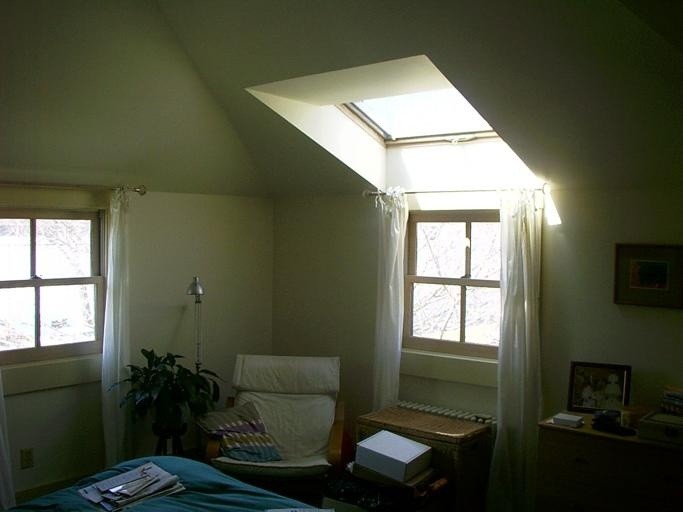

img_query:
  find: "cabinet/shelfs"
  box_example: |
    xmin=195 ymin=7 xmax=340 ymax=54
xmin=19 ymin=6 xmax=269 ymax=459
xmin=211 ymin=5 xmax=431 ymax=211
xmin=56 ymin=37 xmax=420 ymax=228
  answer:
xmin=355 ymin=406 xmax=493 ymax=510
xmin=535 ymin=410 xmax=683 ymax=512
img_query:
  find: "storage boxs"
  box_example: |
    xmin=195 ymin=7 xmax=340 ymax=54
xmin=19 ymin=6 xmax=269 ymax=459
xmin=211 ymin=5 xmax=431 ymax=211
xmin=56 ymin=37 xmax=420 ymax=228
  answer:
xmin=352 ymin=428 xmax=433 ymax=498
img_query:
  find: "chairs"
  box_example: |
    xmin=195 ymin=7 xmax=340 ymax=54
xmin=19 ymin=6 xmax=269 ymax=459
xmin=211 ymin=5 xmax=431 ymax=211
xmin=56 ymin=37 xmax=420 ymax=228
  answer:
xmin=204 ymin=352 xmax=349 ymax=489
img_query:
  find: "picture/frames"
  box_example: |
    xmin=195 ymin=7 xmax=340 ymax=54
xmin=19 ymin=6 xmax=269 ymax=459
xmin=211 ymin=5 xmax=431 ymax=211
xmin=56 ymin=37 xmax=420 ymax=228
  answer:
xmin=613 ymin=243 xmax=682 ymax=311
xmin=566 ymin=361 xmax=631 ymax=415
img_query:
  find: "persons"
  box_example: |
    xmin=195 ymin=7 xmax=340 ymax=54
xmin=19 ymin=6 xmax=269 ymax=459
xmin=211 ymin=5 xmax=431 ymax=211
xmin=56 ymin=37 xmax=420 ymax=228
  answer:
xmin=604 ymin=373 xmax=623 ymax=409
xmin=581 ymin=384 xmax=593 ymax=408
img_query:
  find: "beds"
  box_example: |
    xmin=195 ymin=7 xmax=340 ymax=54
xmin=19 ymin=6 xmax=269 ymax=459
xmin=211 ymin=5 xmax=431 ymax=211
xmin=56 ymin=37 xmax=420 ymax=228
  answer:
xmin=8 ymin=455 xmax=325 ymax=512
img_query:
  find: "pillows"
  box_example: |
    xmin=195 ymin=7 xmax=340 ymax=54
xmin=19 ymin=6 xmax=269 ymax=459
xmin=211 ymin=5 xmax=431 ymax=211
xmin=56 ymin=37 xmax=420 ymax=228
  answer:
xmin=196 ymin=399 xmax=283 ymax=462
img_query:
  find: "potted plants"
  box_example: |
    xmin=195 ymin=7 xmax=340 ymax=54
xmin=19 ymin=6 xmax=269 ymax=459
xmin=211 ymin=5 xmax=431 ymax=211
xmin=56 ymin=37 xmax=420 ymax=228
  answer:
xmin=108 ymin=347 xmax=223 ymax=456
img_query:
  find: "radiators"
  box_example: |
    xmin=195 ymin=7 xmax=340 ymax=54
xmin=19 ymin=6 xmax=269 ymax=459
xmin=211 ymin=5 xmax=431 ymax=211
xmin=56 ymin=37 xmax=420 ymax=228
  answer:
xmin=394 ymin=397 xmax=496 ymax=428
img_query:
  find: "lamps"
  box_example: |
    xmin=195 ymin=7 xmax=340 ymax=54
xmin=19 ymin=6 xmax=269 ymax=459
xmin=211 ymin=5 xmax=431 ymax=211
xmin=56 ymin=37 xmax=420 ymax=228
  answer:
xmin=187 ymin=276 xmax=205 ymax=372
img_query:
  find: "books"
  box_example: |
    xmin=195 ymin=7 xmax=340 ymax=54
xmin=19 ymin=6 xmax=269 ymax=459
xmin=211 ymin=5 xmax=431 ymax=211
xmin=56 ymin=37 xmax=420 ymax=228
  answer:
xmin=76 ymin=459 xmax=186 ymax=511
